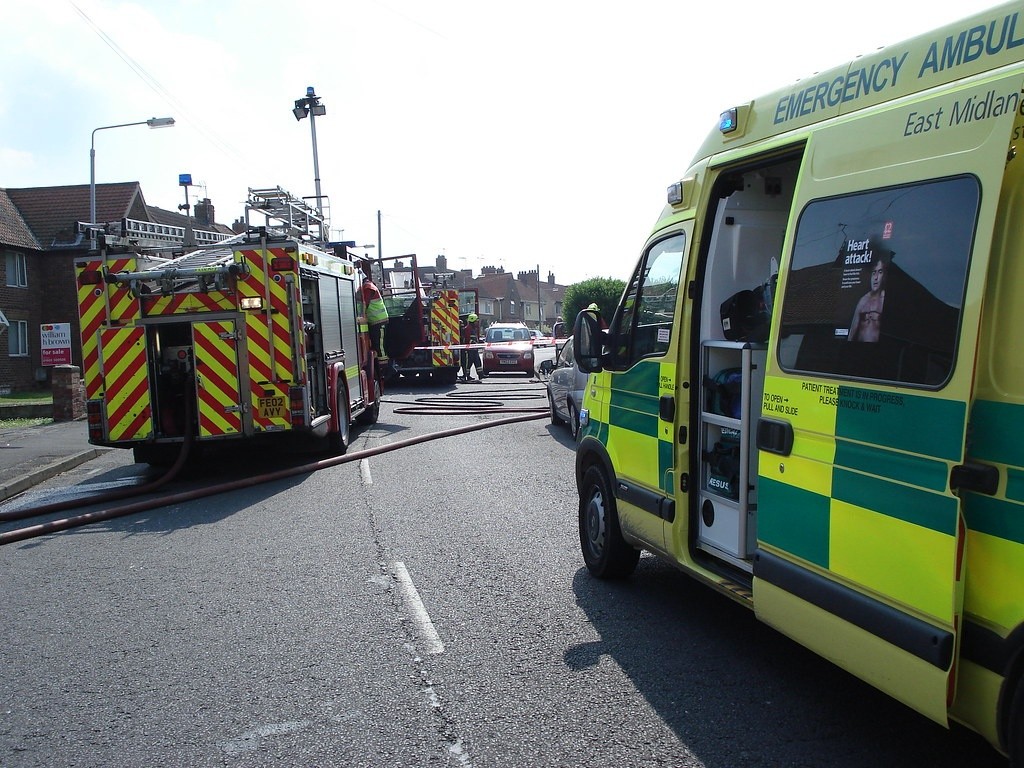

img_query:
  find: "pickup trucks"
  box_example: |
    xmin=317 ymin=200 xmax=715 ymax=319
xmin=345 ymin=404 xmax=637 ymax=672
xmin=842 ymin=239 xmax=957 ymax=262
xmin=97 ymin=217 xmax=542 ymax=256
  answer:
xmin=530 ymin=329 xmax=553 ymax=348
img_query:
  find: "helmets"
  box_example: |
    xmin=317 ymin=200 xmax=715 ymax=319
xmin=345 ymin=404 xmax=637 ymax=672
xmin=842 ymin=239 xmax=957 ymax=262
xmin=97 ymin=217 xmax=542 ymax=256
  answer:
xmin=468 ymin=314 xmax=478 ymax=323
xmin=587 ymin=303 xmax=600 ymax=311
xmin=362 ymin=272 xmax=368 ymax=280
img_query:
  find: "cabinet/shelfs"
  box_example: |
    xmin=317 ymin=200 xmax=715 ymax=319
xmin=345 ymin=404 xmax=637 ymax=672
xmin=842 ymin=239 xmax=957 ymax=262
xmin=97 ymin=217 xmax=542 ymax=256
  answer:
xmin=696 ymin=340 xmax=767 ymax=561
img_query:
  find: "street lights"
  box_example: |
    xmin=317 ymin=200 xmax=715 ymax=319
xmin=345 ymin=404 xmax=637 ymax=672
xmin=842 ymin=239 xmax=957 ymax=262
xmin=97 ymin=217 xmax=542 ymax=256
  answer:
xmin=291 ymin=86 xmax=326 ymax=243
xmin=89 ymin=116 xmax=176 ymax=233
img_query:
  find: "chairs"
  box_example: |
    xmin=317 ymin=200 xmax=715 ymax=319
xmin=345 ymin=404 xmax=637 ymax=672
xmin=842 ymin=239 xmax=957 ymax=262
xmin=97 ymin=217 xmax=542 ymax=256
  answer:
xmin=514 ymin=331 xmax=521 ymax=340
xmin=493 ymin=331 xmax=503 ymax=340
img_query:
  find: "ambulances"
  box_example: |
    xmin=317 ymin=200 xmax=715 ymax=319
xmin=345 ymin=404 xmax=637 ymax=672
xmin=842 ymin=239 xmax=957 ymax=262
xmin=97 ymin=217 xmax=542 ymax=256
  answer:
xmin=573 ymin=1 xmax=1024 ymax=765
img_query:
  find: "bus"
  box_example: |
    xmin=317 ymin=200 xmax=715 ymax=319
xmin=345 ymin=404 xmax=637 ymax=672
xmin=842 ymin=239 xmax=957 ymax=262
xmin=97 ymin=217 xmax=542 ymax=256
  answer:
xmin=551 ymin=316 xmax=567 ymax=349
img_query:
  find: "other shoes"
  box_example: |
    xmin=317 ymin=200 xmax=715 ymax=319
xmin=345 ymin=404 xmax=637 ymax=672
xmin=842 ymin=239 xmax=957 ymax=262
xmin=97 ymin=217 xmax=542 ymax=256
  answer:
xmin=479 ymin=373 xmax=489 ymax=380
xmin=468 ymin=376 xmax=475 ymax=380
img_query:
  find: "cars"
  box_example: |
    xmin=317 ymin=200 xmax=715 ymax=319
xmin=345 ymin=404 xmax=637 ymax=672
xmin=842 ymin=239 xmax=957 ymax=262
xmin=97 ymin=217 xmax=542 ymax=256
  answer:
xmin=538 ymin=328 xmax=608 ymax=441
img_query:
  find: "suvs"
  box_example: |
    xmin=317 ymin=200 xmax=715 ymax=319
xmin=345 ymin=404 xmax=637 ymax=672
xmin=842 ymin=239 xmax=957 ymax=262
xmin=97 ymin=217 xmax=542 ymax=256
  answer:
xmin=483 ymin=321 xmax=535 ymax=379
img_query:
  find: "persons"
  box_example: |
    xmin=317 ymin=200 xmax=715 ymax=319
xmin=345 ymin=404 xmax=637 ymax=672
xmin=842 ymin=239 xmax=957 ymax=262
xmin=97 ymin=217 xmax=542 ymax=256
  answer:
xmin=355 ymin=272 xmax=390 ymax=361
xmin=846 ymin=260 xmax=885 ymax=342
xmin=459 ymin=312 xmax=489 ymax=380
xmin=553 ymin=317 xmax=565 ymax=338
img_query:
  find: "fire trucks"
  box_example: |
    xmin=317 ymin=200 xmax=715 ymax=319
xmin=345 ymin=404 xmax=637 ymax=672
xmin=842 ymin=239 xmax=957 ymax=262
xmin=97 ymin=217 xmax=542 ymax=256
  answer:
xmin=385 ymin=272 xmax=479 ymax=394
xmin=71 ymin=183 xmax=427 ymax=472
